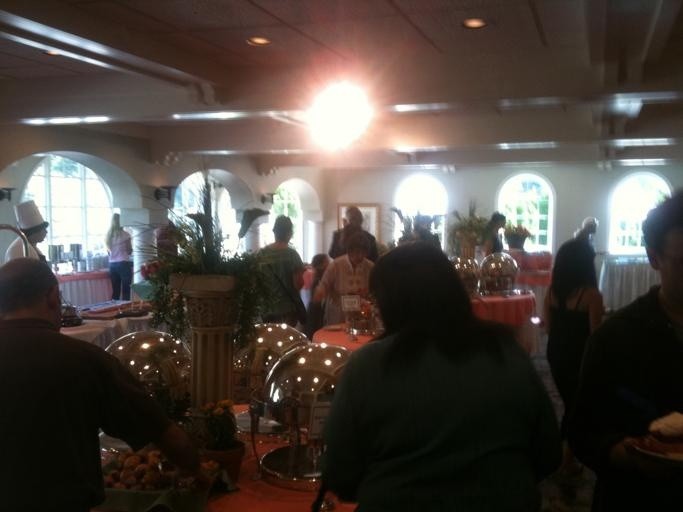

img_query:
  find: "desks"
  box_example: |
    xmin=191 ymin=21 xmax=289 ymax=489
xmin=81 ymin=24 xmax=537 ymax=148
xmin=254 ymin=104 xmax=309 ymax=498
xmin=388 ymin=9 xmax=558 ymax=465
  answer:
xmin=598 ymin=255 xmax=661 ymax=316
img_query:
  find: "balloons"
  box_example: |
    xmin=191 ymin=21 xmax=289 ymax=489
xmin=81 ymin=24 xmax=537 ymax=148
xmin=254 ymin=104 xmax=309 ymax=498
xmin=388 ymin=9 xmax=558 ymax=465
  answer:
xmin=302 ymin=268 xmax=313 ymax=289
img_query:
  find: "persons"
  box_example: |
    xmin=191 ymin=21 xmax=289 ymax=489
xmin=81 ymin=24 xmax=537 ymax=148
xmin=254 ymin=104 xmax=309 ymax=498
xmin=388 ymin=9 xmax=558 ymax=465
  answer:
xmin=310 ymin=253 xmax=328 ymax=301
xmin=312 ymin=231 xmax=375 ymax=326
xmin=4 ymin=221 xmax=50 ymax=263
xmin=329 ymin=207 xmax=379 ymax=262
xmin=153 ymin=220 xmax=188 ymax=260
xmin=475 ymin=212 xmax=507 ymax=267
xmin=574 ymin=217 xmax=598 ymax=239
xmin=561 ymin=186 xmax=682 ymax=511
xmin=399 ymin=215 xmax=441 ymax=248
xmin=256 ymin=215 xmax=305 ymax=327
xmin=324 ymin=238 xmax=580 ymax=511
xmin=0 ymin=256 xmax=200 ymax=511
xmin=106 ymin=213 xmax=135 ymax=300
xmin=540 ymin=235 xmax=606 ymax=478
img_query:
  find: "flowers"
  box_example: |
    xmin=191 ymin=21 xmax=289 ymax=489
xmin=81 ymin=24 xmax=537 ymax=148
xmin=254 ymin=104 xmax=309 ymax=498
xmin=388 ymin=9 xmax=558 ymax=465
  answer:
xmin=503 ymin=225 xmax=533 ymax=240
xmin=444 ymin=199 xmax=490 ymax=256
xmin=118 ymin=157 xmax=280 ymax=345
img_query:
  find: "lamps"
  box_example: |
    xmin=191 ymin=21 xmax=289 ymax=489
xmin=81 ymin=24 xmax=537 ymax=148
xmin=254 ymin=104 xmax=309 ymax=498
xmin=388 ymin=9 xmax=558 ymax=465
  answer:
xmin=152 ymin=183 xmax=177 ymax=200
xmin=258 ymin=191 xmax=277 ymax=205
xmin=0 ymin=186 xmax=16 ymax=199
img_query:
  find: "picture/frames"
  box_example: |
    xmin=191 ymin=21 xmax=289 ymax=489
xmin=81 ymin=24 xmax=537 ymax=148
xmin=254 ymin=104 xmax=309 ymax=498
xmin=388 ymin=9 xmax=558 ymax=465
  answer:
xmin=337 ymin=201 xmax=380 ymax=250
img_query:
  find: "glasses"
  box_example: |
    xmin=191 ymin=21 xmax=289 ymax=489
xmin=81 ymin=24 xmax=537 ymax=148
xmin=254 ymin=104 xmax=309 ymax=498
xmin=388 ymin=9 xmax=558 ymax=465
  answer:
xmin=59 ymin=294 xmax=68 ymax=318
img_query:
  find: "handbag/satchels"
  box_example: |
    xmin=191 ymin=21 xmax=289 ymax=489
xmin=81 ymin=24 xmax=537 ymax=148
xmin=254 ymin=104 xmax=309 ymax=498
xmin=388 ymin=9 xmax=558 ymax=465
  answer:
xmin=295 ymin=297 xmax=307 ymax=324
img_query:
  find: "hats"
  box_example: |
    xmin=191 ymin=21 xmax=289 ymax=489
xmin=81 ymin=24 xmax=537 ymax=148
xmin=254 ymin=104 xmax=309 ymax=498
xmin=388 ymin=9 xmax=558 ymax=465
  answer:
xmin=14 ymin=201 xmax=45 ymax=229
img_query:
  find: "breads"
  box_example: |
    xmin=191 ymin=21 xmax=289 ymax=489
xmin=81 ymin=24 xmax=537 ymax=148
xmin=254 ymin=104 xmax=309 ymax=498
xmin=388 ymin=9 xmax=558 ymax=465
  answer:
xmin=104 ymin=449 xmax=163 ymax=491
xmin=204 ymin=398 xmax=232 ymax=415
